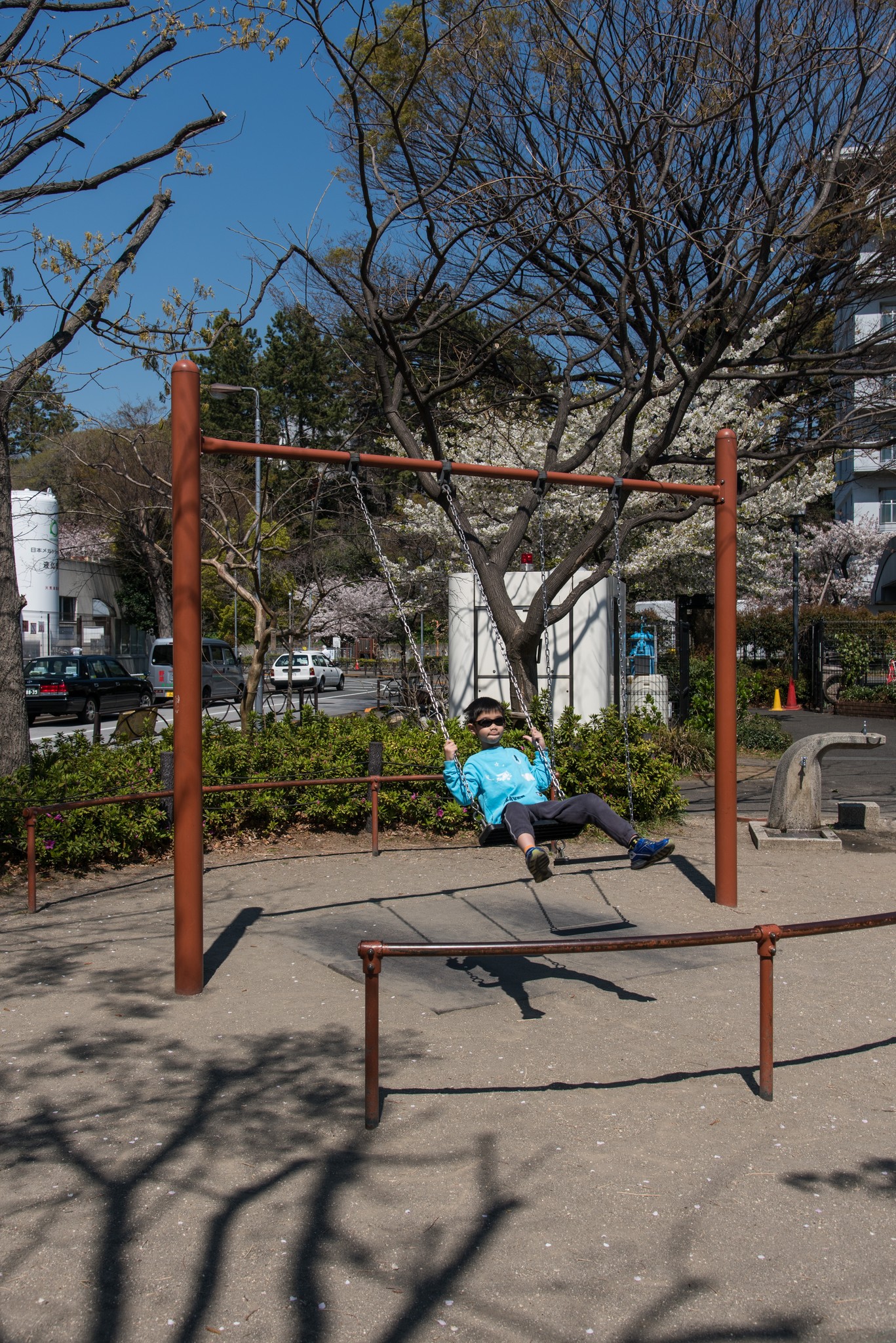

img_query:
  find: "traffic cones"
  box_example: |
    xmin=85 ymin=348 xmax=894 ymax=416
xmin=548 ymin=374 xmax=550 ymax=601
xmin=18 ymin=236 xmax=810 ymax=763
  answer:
xmin=782 ymin=677 xmax=803 ymax=710
xmin=353 ymin=659 xmax=361 ymax=670
xmin=768 ymin=689 xmax=786 ymax=711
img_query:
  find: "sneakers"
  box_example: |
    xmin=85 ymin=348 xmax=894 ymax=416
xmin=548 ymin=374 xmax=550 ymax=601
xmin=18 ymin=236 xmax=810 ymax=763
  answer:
xmin=524 ymin=847 xmax=553 ymax=883
xmin=627 ymin=838 xmax=675 ymax=869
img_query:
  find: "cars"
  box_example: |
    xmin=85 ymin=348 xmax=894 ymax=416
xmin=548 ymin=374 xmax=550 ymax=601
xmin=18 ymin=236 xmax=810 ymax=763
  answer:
xmin=268 ymin=650 xmax=345 ymax=694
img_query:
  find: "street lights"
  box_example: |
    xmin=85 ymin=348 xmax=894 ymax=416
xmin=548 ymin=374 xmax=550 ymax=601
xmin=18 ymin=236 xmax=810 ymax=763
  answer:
xmin=205 ymin=382 xmax=266 ymax=749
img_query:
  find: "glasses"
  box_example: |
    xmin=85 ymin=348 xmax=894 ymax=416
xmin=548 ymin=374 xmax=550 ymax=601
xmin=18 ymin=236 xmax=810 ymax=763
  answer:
xmin=472 ymin=717 xmax=507 ymax=728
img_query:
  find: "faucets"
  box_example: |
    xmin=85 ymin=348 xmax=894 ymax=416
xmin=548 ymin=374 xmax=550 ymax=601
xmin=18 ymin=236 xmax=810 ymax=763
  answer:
xmin=800 ymin=756 xmax=808 ymax=767
xmin=861 ymin=721 xmax=867 ymax=735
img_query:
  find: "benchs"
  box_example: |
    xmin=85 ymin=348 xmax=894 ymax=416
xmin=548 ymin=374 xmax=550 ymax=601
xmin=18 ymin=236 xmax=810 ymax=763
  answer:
xmin=34 ymin=666 xmax=77 ymax=673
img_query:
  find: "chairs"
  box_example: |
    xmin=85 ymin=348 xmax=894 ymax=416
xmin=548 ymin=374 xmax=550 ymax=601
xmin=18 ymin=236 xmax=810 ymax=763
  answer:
xmin=281 ymin=660 xmax=317 ymax=666
xmin=60 ymin=664 xmax=72 ymax=673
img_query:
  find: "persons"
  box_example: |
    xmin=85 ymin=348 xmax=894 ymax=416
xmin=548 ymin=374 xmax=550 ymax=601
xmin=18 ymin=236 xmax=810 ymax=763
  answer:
xmin=443 ymin=698 xmax=675 ymax=884
xmin=322 ymin=645 xmax=331 ymax=659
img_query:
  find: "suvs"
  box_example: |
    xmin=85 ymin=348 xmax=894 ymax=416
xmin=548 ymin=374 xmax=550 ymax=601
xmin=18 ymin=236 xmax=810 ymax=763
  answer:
xmin=22 ymin=654 xmax=156 ymax=727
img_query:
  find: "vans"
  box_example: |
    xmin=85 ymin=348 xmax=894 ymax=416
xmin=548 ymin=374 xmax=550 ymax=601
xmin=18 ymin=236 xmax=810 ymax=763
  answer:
xmin=145 ymin=637 xmax=245 ymax=709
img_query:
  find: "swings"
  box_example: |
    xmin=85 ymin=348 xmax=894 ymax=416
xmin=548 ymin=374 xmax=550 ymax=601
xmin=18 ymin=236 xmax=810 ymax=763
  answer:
xmin=348 ymin=476 xmax=587 ymax=849
xmin=535 ymin=485 xmax=639 ymax=867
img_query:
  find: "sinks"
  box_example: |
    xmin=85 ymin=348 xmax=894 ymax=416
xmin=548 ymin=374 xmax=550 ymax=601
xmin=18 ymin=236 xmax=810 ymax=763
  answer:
xmin=758 ymin=828 xmax=842 ymax=850
